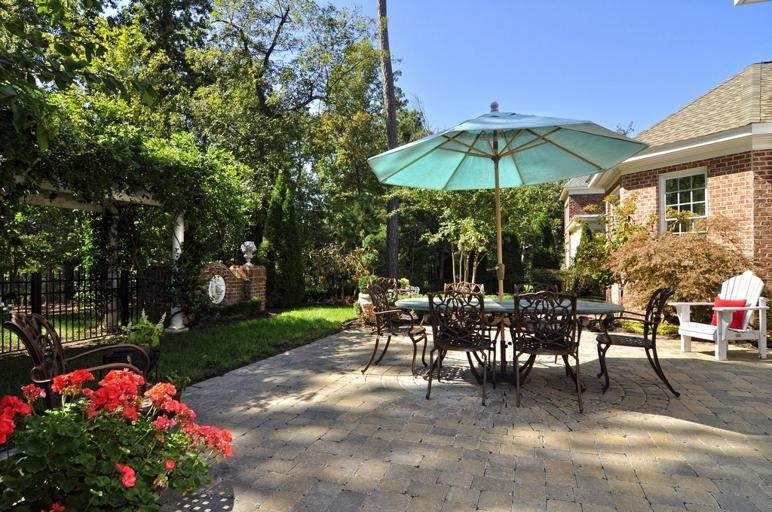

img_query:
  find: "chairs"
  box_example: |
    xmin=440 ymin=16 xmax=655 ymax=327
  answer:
xmin=596 ymin=287 xmax=680 ymax=397
xmin=667 ymin=270 xmax=770 ymax=361
xmin=443 ymin=281 xmax=487 ymax=358
xmin=0 ymin=312 xmax=153 ymax=410
xmin=514 ymin=282 xmax=564 ymax=364
xmin=361 ymin=278 xmax=428 ymax=375
xmin=426 ymin=291 xmax=501 ymax=406
xmin=510 ymin=291 xmax=584 ymax=413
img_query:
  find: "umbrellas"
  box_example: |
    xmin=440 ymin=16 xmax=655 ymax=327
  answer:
xmin=372 ymin=99 xmax=650 ymax=376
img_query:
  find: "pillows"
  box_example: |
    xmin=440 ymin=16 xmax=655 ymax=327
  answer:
xmin=710 ymin=297 xmax=746 ymax=329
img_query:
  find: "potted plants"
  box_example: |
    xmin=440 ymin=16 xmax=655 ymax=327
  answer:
xmin=358 ymin=275 xmax=379 ymax=317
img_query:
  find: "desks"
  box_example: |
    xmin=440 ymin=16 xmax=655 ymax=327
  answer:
xmin=395 ymin=296 xmax=624 ymax=386
